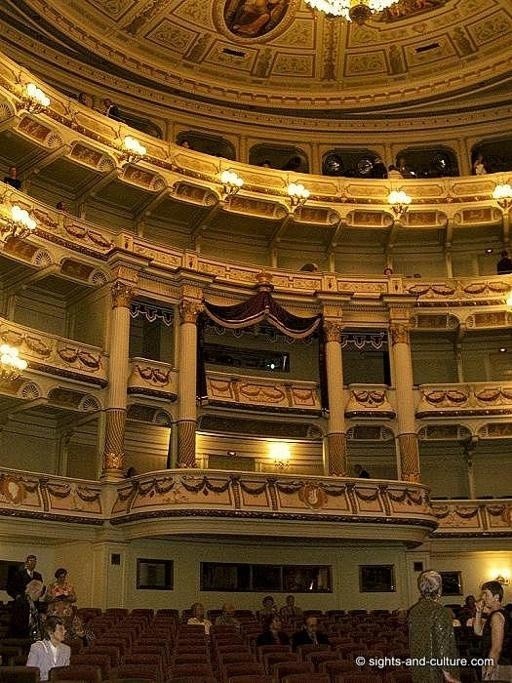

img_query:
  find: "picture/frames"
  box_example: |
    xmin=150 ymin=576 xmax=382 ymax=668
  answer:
xmin=438 ymin=571 xmax=462 ymax=597
xmin=136 ymin=558 xmax=174 ymax=589
xmin=359 ymin=564 xmax=395 ymax=592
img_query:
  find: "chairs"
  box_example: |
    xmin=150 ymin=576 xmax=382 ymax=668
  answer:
xmin=0 ymin=604 xmax=463 ymax=683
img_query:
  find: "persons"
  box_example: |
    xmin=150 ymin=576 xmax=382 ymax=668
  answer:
xmin=67 ymin=613 xmax=96 ymax=646
xmin=465 ymin=580 xmax=512 ymax=683
xmin=292 ymin=615 xmax=331 ymax=645
xmin=408 ymin=569 xmax=464 ymax=683
xmin=188 ymin=602 xmax=213 ymax=634
xmin=215 ymin=603 xmax=242 ymax=625
xmin=26 ymin=615 xmax=71 ymax=681
xmin=7 ymin=554 xmax=45 ymax=639
xmin=256 ymin=616 xmax=290 ymax=645
xmin=257 ymin=595 xmax=278 ymax=615
xmin=44 ymin=568 xmax=78 ymax=622
xmin=280 ymin=594 xmax=303 ymax=616
